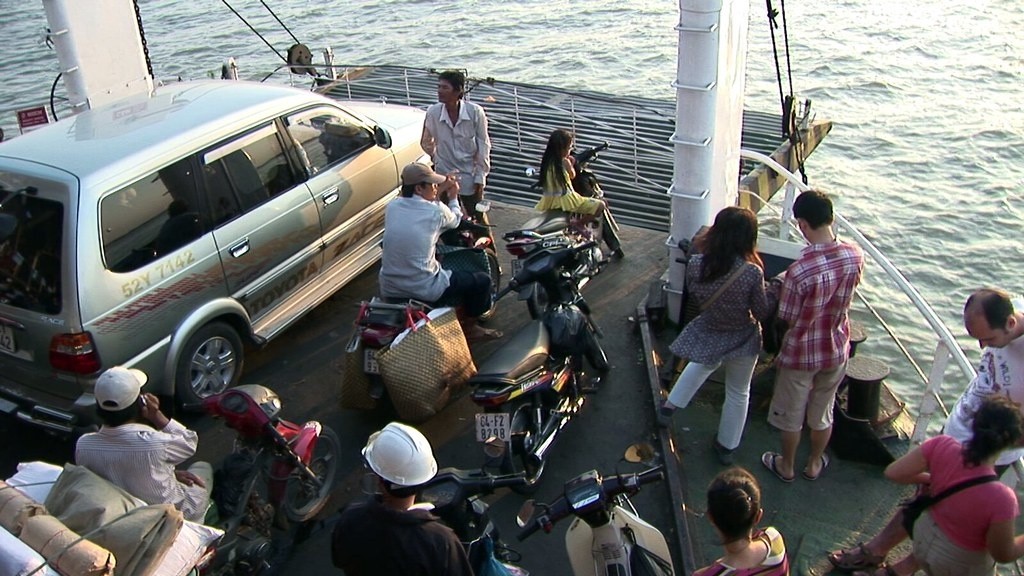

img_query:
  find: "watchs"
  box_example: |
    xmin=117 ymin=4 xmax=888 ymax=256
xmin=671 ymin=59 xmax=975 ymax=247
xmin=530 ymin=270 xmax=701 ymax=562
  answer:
xmin=884 ymin=393 xmax=1024 ymax=576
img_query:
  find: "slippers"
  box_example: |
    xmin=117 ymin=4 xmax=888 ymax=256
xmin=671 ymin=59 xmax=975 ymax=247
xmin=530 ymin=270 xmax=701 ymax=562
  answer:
xmin=468 ymin=326 xmax=503 ymax=342
xmin=761 ymin=451 xmax=796 ymax=482
xmin=803 ymin=451 xmax=830 ymax=480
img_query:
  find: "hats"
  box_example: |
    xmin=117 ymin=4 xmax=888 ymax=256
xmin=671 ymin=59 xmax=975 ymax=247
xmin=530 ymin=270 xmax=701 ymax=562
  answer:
xmin=95 ymin=365 xmax=149 ymax=409
xmin=402 ymin=162 xmax=447 ymax=186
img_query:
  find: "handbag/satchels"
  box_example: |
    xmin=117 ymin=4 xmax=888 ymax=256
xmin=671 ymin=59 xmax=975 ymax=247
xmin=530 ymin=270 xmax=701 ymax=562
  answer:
xmin=376 ymin=305 xmax=477 ymax=424
xmin=337 ymin=301 xmax=376 ymax=410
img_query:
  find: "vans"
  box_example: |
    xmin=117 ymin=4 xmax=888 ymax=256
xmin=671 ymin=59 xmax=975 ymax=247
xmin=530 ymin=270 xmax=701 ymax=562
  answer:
xmin=0 ymin=76 xmax=436 ymax=445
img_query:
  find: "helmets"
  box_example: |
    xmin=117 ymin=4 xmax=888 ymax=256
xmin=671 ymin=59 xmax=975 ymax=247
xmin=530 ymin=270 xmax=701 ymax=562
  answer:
xmin=361 ymin=421 xmax=438 ymax=486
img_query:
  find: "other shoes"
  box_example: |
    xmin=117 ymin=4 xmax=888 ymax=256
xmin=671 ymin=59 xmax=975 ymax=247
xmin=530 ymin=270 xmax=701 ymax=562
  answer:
xmin=571 ymin=218 xmax=592 ymax=240
xmin=710 ymin=429 xmax=732 ymax=457
xmin=656 ymin=396 xmax=676 ymax=419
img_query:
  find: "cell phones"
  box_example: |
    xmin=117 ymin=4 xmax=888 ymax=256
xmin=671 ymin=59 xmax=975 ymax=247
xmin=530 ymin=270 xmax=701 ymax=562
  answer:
xmin=139 ymin=395 xmax=147 ymax=406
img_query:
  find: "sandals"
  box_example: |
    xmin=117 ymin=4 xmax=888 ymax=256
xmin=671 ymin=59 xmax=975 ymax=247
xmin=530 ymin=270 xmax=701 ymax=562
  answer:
xmin=827 ymin=541 xmax=898 ymax=576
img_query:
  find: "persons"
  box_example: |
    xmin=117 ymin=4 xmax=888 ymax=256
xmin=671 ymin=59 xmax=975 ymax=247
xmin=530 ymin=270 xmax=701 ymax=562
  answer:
xmin=829 ymin=285 xmax=1024 ymax=570
xmin=74 ymin=364 xmax=213 ymax=523
xmin=694 ymin=467 xmax=790 ymax=576
xmin=762 ymin=190 xmax=864 ymax=483
xmin=329 ymin=421 xmax=474 ymax=576
xmin=378 ymin=161 xmax=504 ymax=340
xmin=533 ymin=128 xmax=605 ymax=240
xmin=420 ymin=71 xmax=505 ymax=276
xmin=657 ymin=206 xmax=769 ymax=455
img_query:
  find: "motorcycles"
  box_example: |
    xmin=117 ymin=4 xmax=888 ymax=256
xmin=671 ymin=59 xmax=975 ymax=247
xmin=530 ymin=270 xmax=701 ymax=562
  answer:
xmin=0 ymin=383 xmax=340 ymax=576
xmin=355 ymin=465 xmax=532 ymax=576
xmin=512 ymin=440 xmax=677 ymax=576
xmin=501 ymin=140 xmax=625 ymax=320
xmin=468 ymin=238 xmax=613 ymax=496
xmin=345 ymin=198 xmax=504 ymax=409
